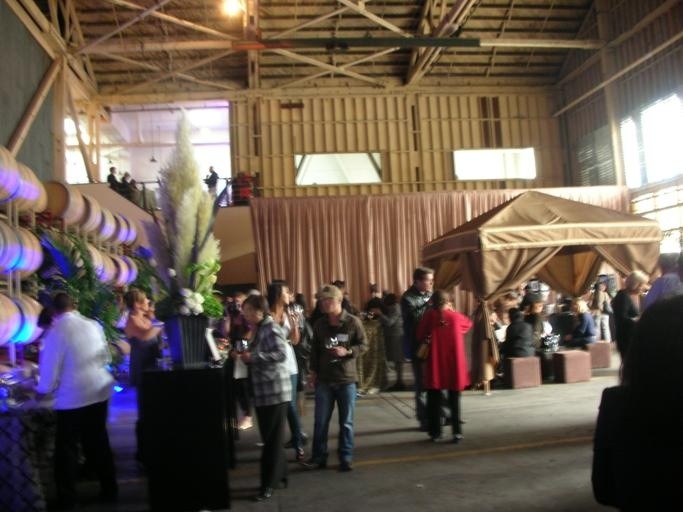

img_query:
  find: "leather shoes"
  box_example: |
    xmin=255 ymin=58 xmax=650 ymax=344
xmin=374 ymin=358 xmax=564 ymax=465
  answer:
xmin=295 ymin=447 xmax=305 ymax=459
xmin=255 ymin=489 xmax=269 ymax=501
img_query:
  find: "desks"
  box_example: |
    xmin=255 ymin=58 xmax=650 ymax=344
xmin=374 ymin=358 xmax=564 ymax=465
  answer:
xmin=137 ymin=340 xmax=237 ymax=511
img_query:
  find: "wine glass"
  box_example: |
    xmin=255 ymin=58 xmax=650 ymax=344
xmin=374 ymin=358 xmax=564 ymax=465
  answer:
xmin=286 ymin=302 xmax=302 ymax=331
xmin=323 ymin=335 xmax=341 ymax=365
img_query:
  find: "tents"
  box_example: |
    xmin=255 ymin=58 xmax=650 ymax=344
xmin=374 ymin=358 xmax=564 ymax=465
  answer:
xmin=424 ymin=190 xmax=663 ymax=392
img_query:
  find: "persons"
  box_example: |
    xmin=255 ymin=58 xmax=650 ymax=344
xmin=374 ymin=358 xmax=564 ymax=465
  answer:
xmin=32 ymin=304 xmax=60 ymax=467
xmin=610 ymin=269 xmax=650 ymax=356
xmin=123 ymin=288 xmax=163 ymax=463
xmin=212 ymin=266 xmax=474 ymax=499
xmin=203 ymin=166 xmax=219 ymax=198
xmin=144 ymin=298 xmax=167 ymax=371
xmin=592 ymin=294 xmax=683 ymax=509
xmin=642 ymin=252 xmax=683 ymax=310
xmin=120 ymin=172 xmax=131 ymax=187
xmin=488 ymin=284 xmax=614 ymax=358
xmin=129 ymin=178 xmax=138 ymax=190
xmin=35 ymin=290 xmax=118 ymax=511
xmin=106 ymin=167 xmax=119 ymax=192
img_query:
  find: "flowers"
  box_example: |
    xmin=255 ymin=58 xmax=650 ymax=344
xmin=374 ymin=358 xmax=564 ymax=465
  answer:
xmin=130 ymin=100 xmax=228 ymax=319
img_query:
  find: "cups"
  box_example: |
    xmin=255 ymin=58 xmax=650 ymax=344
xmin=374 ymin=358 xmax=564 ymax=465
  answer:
xmin=358 ymin=311 xmax=374 ymax=320
xmin=233 ymin=339 xmax=248 ymax=356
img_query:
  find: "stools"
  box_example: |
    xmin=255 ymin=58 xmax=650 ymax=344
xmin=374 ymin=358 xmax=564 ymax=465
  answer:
xmin=552 ymin=350 xmax=593 ymax=384
xmin=585 ymin=340 xmax=613 ymax=369
xmin=504 ymin=356 xmax=543 ymax=389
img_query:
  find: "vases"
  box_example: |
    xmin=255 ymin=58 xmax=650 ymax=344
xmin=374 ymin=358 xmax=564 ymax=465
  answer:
xmin=162 ymin=313 xmax=209 ymax=371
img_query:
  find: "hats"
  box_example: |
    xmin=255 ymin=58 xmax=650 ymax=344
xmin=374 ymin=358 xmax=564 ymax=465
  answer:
xmin=317 ymin=285 xmax=344 ymax=300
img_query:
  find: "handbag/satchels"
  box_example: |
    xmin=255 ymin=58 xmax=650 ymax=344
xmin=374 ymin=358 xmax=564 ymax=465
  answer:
xmin=417 ymin=342 xmax=430 ymax=360
xmin=234 ymin=339 xmax=299 ymax=380
xmin=542 ymin=335 xmax=560 ymax=353
